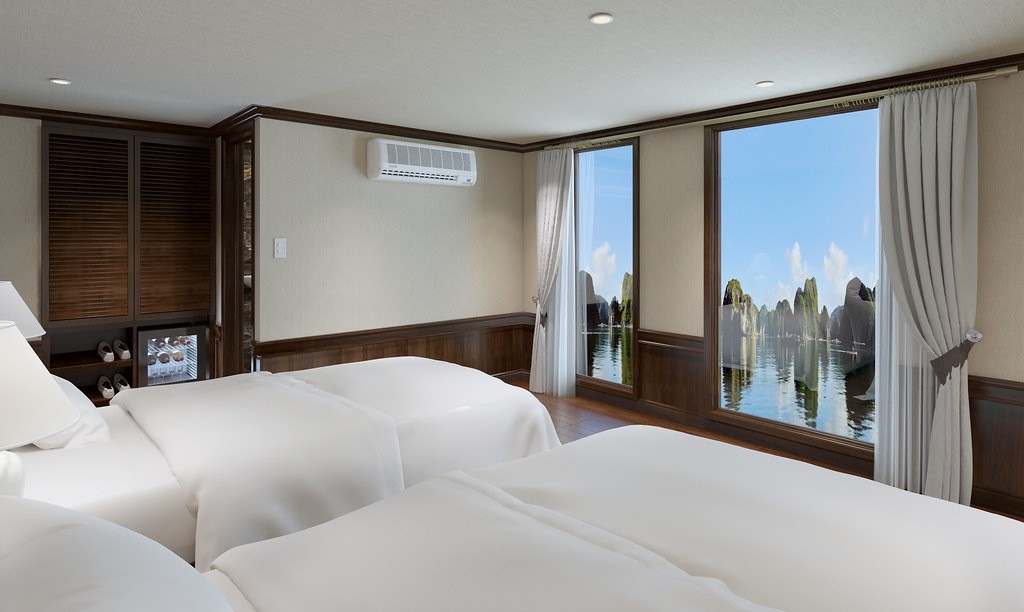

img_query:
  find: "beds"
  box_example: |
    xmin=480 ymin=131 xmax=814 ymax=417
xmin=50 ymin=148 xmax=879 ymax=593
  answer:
xmin=0 ymin=355 xmax=1024 ymax=612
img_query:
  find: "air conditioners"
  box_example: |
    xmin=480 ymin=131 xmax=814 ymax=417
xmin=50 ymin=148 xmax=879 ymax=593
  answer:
xmin=365 ymin=137 xmax=478 ymax=189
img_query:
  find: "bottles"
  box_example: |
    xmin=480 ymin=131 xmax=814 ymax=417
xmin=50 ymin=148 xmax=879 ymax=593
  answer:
xmin=147 ymin=336 xmax=191 ymax=366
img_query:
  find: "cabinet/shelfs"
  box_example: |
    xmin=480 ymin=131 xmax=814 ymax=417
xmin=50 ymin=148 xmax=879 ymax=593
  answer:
xmin=42 ymin=122 xmax=214 ymax=406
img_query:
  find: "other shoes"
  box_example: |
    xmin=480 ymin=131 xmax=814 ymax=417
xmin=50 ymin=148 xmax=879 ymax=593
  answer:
xmin=113 ymin=374 xmax=130 ymax=391
xmin=113 ymin=339 xmax=131 ymax=360
xmin=97 ymin=376 xmax=114 ymax=398
xmin=98 ymin=340 xmax=114 ymax=362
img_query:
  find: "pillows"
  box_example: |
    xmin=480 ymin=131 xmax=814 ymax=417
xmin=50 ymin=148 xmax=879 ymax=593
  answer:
xmin=10 ymin=491 xmax=229 ymax=612
xmin=30 ymin=372 xmax=106 ymax=452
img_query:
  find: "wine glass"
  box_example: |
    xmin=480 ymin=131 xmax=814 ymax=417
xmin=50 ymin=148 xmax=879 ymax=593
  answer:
xmin=152 ymin=366 xmax=183 ymax=382
